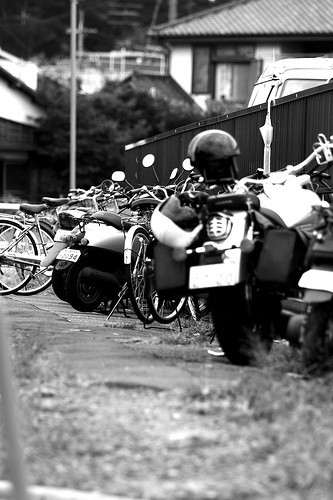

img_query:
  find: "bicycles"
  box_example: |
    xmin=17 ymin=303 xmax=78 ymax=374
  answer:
xmin=0 ymin=153 xmax=134 ymax=298
xmin=98 ymin=168 xmax=206 ymax=331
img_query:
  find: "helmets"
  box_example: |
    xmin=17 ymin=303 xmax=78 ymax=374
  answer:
xmin=187 ymin=129 xmax=241 ymax=165
xmin=149 ymin=193 xmax=203 ymax=248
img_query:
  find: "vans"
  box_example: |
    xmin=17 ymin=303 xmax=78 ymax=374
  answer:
xmin=246 ymin=56 xmax=333 ymax=109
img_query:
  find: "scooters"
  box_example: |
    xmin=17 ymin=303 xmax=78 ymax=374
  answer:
xmin=38 ymin=150 xmax=195 ymax=314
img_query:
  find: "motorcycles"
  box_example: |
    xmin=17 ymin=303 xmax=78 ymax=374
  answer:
xmin=147 ymin=130 xmax=333 ymax=379
